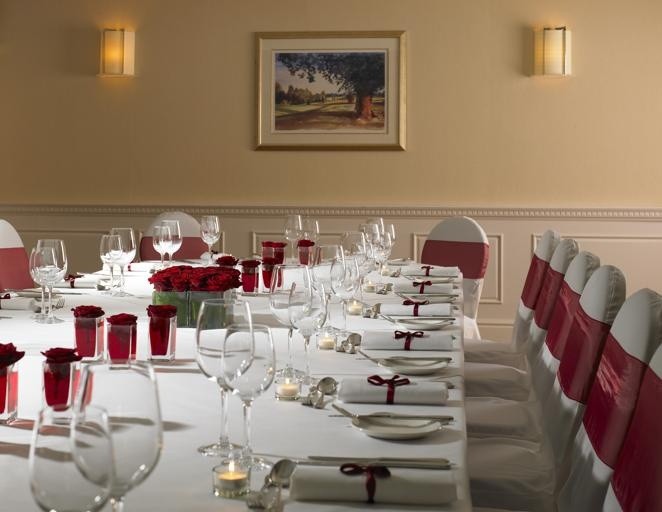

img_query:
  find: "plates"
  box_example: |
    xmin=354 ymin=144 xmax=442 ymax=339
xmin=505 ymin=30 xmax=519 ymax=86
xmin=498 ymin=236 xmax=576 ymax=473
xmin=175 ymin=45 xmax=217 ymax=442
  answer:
xmin=16 ymin=288 xmax=61 ymax=298
xmin=351 ymin=292 xmax=456 ymax=442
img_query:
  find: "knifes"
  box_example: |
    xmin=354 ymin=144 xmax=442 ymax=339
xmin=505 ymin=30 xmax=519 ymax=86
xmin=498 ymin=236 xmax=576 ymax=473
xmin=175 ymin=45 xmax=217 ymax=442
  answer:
xmin=296 ymin=453 xmax=457 ymax=473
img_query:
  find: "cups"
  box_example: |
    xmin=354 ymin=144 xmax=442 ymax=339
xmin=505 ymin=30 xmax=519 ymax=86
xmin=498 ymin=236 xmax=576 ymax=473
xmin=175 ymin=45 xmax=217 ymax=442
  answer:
xmin=261 ymin=264 xmax=279 ymax=293
xmin=26 ymin=402 xmax=117 ymax=512
xmin=261 ymin=247 xmax=274 ymax=264
xmin=40 ymin=360 xmax=81 ymax=412
xmin=73 ymin=313 xmax=177 ymax=371
xmin=242 ymin=266 xmax=258 ymax=294
xmin=0 ymin=361 xmax=19 ymax=427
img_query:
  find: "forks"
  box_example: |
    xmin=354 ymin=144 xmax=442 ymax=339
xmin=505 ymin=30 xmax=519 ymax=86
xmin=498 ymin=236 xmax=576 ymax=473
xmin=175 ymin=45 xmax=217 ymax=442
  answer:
xmin=327 ymin=402 xmax=456 ymax=422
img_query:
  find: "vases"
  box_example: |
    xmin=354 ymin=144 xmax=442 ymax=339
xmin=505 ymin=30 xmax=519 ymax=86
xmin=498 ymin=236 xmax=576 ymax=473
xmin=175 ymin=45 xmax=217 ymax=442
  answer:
xmin=190 ymin=290 xmax=235 ymax=330
xmin=152 ymin=289 xmax=192 ymax=328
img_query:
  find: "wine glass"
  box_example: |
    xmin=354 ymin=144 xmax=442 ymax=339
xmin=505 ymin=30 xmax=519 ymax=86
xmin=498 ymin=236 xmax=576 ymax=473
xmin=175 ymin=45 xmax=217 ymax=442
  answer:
xmin=69 ymin=357 xmax=164 ymax=512
xmin=199 ymin=214 xmax=221 ymax=267
xmin=268 ymin=211 xmax=398 ymax=390
xmin=151 ymin=220 xmax=182 ymax=272
xmin=97 ymin=226 xmax=137 ymax=298
xmin=191 ymin=296 xmax=279 ymax=472
xmin=26 ymin=238 xmax=68 ymax=325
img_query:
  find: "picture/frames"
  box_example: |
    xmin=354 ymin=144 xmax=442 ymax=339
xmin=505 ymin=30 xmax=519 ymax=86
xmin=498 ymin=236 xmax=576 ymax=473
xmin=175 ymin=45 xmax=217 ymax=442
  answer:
xmin=252 ymin=30 xmax=406 ymax=151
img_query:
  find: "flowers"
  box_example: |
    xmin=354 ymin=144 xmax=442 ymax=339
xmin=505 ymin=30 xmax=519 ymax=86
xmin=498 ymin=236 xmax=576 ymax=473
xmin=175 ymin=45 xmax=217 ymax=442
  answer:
xmin=190 ymin=266 xmax=242 ymax=330
xmin=216 ymin=240 xmax=315 ymax=289
xmin=0 ymin=305 xmax=179 ymax=394
xmin=148 ymin=265 xmax=192 ymax=327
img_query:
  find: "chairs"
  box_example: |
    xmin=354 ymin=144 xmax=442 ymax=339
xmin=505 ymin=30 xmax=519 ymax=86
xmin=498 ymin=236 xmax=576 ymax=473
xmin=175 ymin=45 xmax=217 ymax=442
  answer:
xmin=421 ymin=213 xmax=490 ymax=341
xmin=1 ymin=219 xmax=34 ymax=292
xmin=139 ymin=211 xmax=208 ymax=260
xmin=466 ymin=263 xmax=628 ymax=510
xmin=464 ymin=239 xmax=580 ymax=401
xmin=463 ymin=249 xmax=599 ymax=441
xmin=474 ymin=283 xmax=661 ymax=510
xmin=462 ymin=230 xmax=560 ymax=371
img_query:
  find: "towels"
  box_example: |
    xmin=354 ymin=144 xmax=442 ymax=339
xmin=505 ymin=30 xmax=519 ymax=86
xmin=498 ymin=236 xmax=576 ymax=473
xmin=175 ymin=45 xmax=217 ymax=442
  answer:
xmin=1 ymin=298 xmax=34 ymax=310
xmin=104 ymin=263 xmax=145 ymax=272
xmin=200 ymin=249 xmax=231 ymax=263
xmin=52 ymin=277 xmax=99 ymax=289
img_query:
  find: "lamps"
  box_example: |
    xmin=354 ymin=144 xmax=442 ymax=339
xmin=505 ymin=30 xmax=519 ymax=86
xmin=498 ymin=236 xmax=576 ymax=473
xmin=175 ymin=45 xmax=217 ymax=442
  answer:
xmin=532 ymin=27 xmax=573 ymax=78
xmin=99 ymin=29 xmax=136 ymax=78
